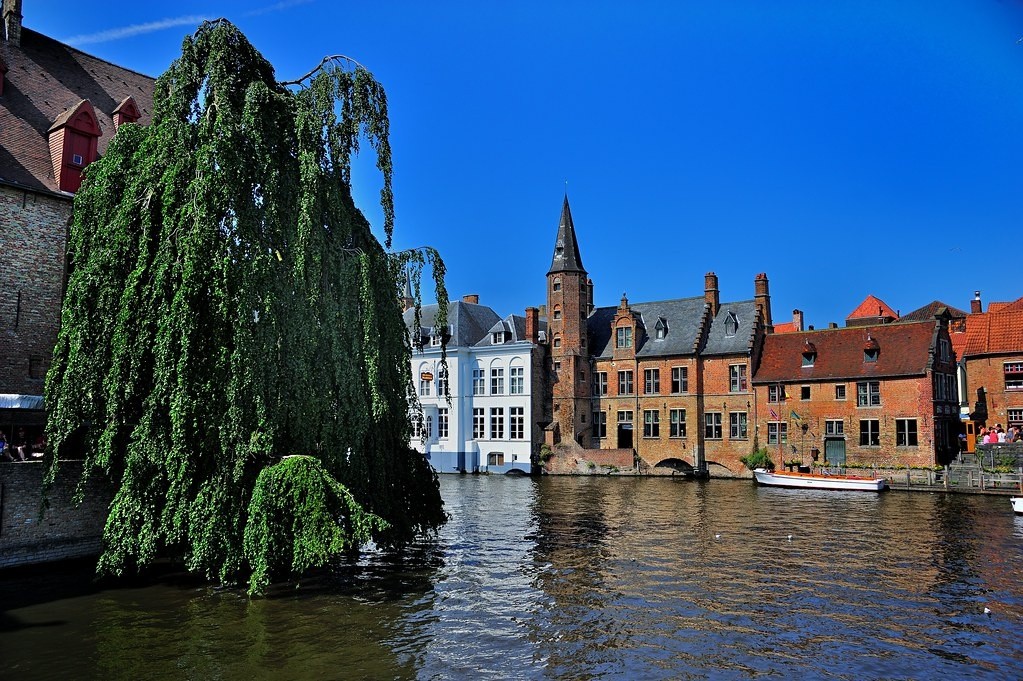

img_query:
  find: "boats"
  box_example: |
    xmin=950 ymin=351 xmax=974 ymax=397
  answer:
xmin=755 ymin=385 xmax=885 ymax=493
xmin=1009 ymin=497 xmax=1023 ymax=514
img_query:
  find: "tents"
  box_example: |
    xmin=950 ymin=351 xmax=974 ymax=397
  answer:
xmin=0 ymin=394 xmax=45 ymax=444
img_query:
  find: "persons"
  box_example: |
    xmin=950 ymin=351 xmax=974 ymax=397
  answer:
xmin=959 ymin=430 xmax=967 ymax=450
xmin=0 ymin=426 xmax=47 ymax=462
xmin=978 ymin=423 xmax=1023 ymax=444
